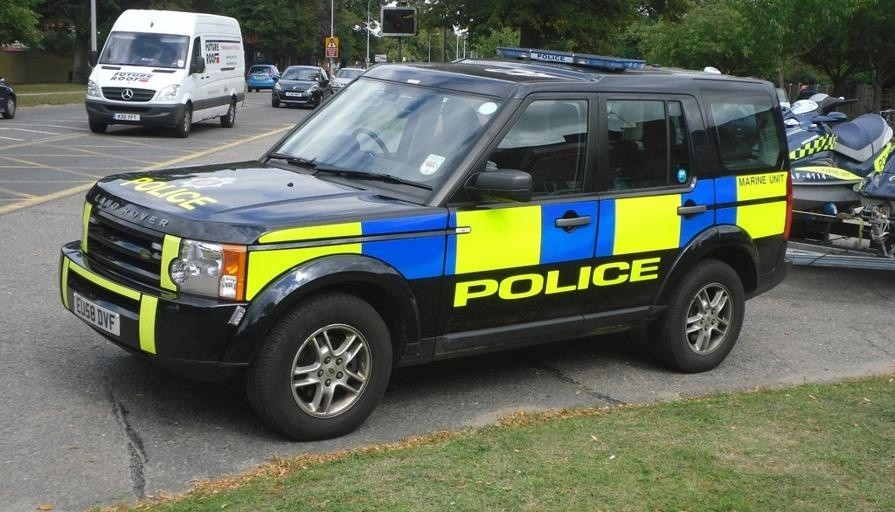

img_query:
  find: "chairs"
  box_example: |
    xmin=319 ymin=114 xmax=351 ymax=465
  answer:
xmin=522 ymin=104 xmax=676 ymax=189
xmin=409 ymin=98 xmax=483 ymax=180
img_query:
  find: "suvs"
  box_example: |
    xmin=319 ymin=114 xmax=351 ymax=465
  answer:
xmin=56 ymin=45 xmax=795 ymax=441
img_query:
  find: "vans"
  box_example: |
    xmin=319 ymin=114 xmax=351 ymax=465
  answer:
xmin=84 ymin=5 xmax=248 ymax=139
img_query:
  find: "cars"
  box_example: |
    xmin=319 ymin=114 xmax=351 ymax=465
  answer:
xmin=271 ymin=64 xmax=333 ymax=110
xmin=247 ymin=63 xmax=281 ymax=92
xmin=0 ymin=76 xmax=17 ymax=119
xmin=330 ymin=67 xmax=368 ymax=95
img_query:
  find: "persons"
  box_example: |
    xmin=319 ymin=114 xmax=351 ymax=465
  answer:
xmin=796 ymin=80 xmax=817 ymax=99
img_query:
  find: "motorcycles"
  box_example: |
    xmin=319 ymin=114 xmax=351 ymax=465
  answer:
xmin=717 ymin=86 xmax=894 ymax=257
